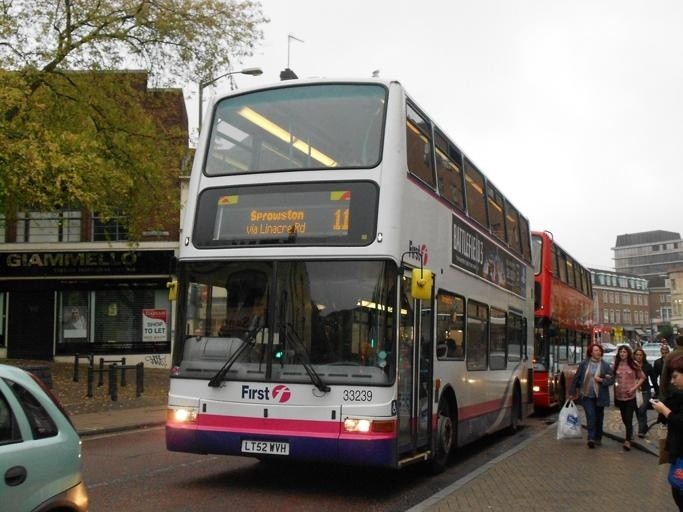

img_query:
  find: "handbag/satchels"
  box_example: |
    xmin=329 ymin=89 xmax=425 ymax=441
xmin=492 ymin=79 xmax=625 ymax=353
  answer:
xmin=635 ymin=390 xmax=644 ymax=407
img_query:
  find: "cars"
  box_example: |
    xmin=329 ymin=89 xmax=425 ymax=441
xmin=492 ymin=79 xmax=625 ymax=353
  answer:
xmin=0 ymin=366 xmax=89 ymax=510
xmin=599 ymin=340 xmax=673 ymax=367
xmin=507 ymin=343 xmax=585 ymax=374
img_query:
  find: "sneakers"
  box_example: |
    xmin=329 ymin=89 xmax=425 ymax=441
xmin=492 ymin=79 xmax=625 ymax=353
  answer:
xmin=588 ymin=440 xmax=601 ymax=449
xmin=623 ymin=440 xmax=630 ymax=450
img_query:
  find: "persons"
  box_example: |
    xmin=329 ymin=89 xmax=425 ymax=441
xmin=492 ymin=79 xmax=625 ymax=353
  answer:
xmin=648 ymin=364 xmax=682 ymax=512
xmin=604 ymin=344 xmax=647 ymax=451
xmin=630 ymin=348 xmax=660 ymax=441
xmin=653 ymin=342 xmax=672 ymax=382
xmin=567 ymin=343 xmax=616 ymax=449
xmin=658 ymin=334 xmax=682 ymax=400
xmin=65 ymin=306 xmax=87 ymax=331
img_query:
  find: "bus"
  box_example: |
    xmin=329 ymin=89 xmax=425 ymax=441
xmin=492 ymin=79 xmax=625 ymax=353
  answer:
xmin=529 ymin=231 xmax=597 ymax=413
xmin=160 ymin=78 xmax=537 ymax=492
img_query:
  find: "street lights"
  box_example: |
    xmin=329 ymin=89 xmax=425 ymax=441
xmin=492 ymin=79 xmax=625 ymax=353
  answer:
xmin=286 ymin=36 xmax=304 ymax=70
xmin=197 ymin=67 xmax=262 ymax=140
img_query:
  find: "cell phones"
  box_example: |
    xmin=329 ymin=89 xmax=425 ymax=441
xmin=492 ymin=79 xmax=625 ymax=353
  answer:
xmin=649 ymin=398 xmax=656 ymax=404
